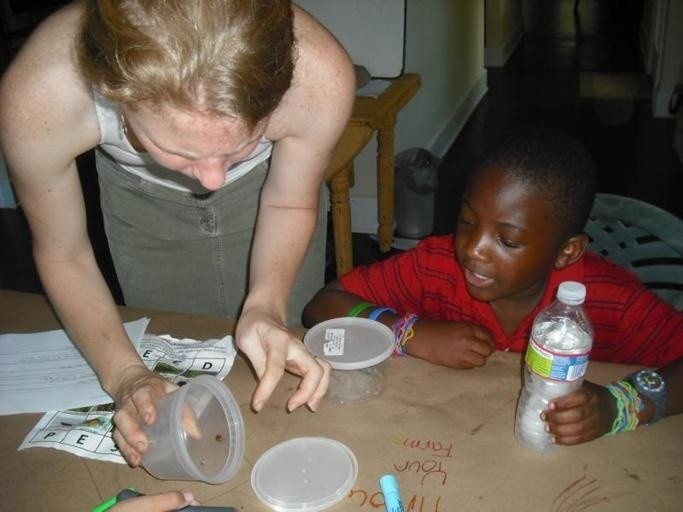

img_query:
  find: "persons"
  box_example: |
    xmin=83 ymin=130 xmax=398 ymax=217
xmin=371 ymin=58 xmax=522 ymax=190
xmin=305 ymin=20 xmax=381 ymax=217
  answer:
xmin=1 ymin=1 xmax=357 ymax=467
xmin=105 ymin=490 xmax=203 ymax=512
xmin=302 ymin=109 xmax=683 ymax=447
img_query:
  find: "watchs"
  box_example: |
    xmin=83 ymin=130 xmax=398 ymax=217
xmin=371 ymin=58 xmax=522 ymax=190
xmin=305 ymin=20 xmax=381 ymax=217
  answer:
xmin=624 ymin=368 xmax=668 ymax=427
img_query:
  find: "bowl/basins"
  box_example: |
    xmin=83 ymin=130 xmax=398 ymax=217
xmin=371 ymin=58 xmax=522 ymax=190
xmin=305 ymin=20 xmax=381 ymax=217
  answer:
xmin=515 ymin=281 xmax=596 ymax=457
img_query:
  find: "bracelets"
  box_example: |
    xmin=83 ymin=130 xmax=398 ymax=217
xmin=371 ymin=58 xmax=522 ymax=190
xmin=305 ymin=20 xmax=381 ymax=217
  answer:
xmin=391 ymin=312 xmax=418 ymax=357
xmin=349 ymin=302 xmax=375 ymax=319
xmin=368 ymin=305 xmax=397 ymax=321
xmin=606 ymin=381 xmax=644 ymax=436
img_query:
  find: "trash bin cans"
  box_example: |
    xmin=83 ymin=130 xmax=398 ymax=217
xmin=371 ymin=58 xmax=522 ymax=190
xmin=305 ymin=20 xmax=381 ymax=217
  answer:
xmin=395 ymin=147 xmax=444 ymax=238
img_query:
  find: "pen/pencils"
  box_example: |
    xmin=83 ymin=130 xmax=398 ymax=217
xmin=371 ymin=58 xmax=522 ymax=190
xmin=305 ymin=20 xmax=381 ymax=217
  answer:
xmin=379 ymin=474 xmax=404 ymax=512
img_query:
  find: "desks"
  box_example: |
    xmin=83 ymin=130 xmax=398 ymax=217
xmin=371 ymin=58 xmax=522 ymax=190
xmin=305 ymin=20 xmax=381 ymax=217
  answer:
xmin=1 ymin=287 xmax=683 ymax=510
